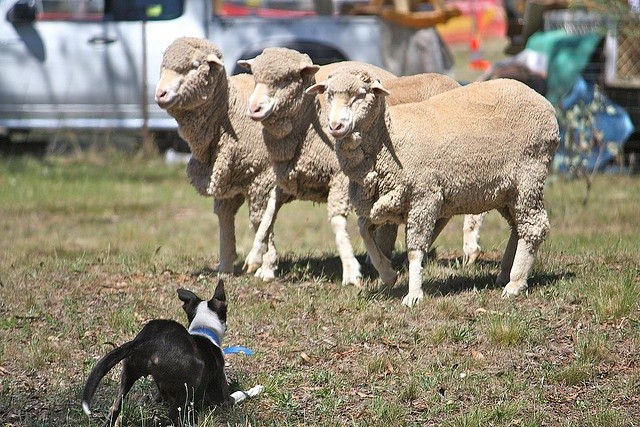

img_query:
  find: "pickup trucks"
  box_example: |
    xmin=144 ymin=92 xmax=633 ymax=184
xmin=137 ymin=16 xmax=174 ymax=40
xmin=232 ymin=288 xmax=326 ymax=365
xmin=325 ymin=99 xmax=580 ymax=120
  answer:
xmin=0 ymin=0 xmax=383 ymax=140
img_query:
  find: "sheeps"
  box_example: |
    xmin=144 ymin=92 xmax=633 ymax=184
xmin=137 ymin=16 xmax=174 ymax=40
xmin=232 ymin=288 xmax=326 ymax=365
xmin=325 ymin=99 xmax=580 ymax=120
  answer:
xmin=154 ymin=34 xmax=401 ymax=283
xmin=237 ymin=45 xmax=489 ymax=288
xmin=305 ymin=65 xmax=562 ymax=308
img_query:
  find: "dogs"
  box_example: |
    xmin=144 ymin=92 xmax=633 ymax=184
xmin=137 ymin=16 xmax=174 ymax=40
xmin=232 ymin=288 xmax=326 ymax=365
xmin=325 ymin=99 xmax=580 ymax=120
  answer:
xmin=82 ymin=277 xmax=266 ymax=427
